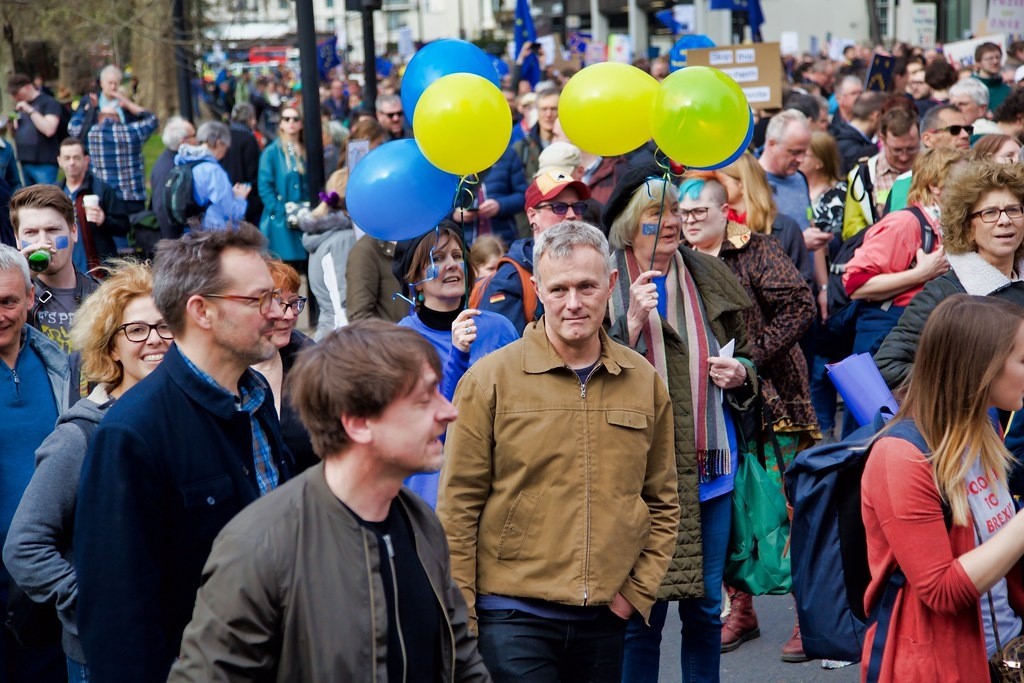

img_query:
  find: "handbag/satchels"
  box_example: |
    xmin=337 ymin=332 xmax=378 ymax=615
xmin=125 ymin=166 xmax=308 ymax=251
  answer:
xmin=990 ymin=637 xmax=1024 ymax=682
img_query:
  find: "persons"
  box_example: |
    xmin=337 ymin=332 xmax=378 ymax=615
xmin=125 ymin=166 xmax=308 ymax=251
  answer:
xmin=0 ymin=41 xmax=1024 ymax=683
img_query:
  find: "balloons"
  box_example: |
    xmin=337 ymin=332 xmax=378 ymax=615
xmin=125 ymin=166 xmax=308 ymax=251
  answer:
xmin=400 ymin=38 xmax=502 ymax=126
xmin=412 ymin=73 xmax=512 ymax=176
xmin=650 ymin=66 xmax=750 ymax=167
xmin=558 ymin=61 xmax=659 ymax=156
xmin=686 ymin=106 xmax=755 ymax=169
xmin=346 ymin=138 xmax=461 ymax=242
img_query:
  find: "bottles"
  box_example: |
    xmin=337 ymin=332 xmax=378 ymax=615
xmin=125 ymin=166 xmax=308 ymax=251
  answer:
xmin=28 ymin=249 xmax=52 ymax=272
xmin=8 ymin=110 xmax=18 ymax=122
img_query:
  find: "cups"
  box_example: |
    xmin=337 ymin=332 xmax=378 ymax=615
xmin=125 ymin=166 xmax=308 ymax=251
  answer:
xmin=83 ymin=194 xmax=100 ymax=222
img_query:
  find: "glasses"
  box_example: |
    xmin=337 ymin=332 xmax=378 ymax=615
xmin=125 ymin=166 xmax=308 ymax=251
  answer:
xmin=279 ymin=295 xmax=308 ymax=315
xmin=380 ymin=111 xmax=404 ymax=118
xmin=932 ymin=125 xmax=974 ymax=136
xmin=967 ymin=204 xmax=1024 ymax=223
xmin=110 ymin=322 xmax=174 ymax=342
xmin=536 ymin=201 xmax=589 ymax=216
xmin=281 ymin=116 xmax=302 ymax=122
xmin=884 ymin=143 xmax=919 ymax=156
xmin=185 ymin=287 xmax=282 ymax=315
xmin=679 ymin=205 xmax=722 ymax=222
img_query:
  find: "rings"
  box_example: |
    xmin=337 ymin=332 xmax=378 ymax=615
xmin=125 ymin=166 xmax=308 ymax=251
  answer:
xmin=466 ymin=327 xmax=471 ymax=334
xmin=464 ymin=321 xmax=469 ymax=327
xmin=715 ymin=372 xmax=719 ymax=380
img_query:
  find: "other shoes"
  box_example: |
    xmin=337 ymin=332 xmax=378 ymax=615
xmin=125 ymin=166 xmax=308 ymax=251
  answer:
xmin=819 ymin=427 xmax=837 ymax=444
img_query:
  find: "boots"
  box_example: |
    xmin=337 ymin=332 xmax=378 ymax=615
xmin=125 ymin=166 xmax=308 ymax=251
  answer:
xmin=719 ymin=586 xmax=761 ymax=648
xmin=781 ymin=592 xmax=807 ymax=661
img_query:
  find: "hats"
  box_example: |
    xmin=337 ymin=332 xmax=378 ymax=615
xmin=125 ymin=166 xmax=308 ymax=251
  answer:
xmin=524 ymin=172 xmax=591 ymax=213
xmin=5 ymin=74 xmax=31 ymax=93
xmin=392 ymin=220 xmax=473 ymax=297
xmin=532 ymin=143 xmax=581 ymax=178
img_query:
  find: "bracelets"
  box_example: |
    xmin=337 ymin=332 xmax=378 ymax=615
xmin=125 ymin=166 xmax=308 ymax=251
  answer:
xmin=819 ymin=285 xmax=827 ymax=290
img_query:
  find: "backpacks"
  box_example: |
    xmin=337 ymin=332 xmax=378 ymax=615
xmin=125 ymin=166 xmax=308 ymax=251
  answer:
xmin=823 ymin=206 xmax=936 ymax=338
xmin=468 ymin=257 xmax=539 ymax=323
xmin=164 ymin=160 xmax=212 ymax=227
xmin=782 ymin=405 xmax=952 ymax=661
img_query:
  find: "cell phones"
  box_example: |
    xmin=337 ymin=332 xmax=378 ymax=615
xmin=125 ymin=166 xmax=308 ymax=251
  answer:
xmin=530 ymin=43 xmax=540 ymax=49
xmin=467 ymin=208 xmax=480 ymax=211
